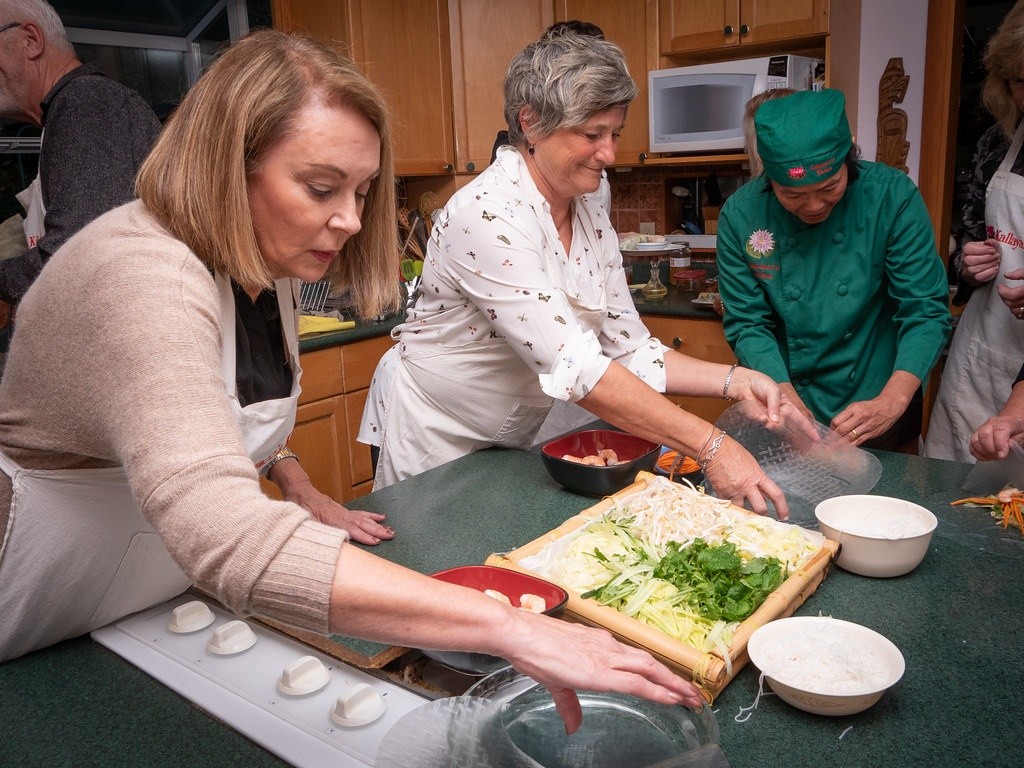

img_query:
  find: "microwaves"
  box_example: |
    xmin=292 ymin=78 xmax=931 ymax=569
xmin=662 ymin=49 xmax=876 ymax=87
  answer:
xmin=648 ymin=53 xmax=824 ymax=154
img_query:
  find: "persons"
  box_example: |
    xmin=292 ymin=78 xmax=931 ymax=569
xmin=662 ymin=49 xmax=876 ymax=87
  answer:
xmin=356 ymin=32 xmax=821 ymax=521
xmin=0 ymin=0 xmax=164 ymax=305
xmin=0 ymin=30 xmax=702 ymax=735
xmin=923 ymin=0 xmax=1024 ymax=463
xmin=968 ymin=363 xmax=1024 ymax=461
xmin=716 ymin=88 xmax=953 ymax=456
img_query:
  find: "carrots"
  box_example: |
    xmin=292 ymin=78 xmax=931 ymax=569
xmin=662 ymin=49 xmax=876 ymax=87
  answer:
xmin=658 ymin=449 xmax=702 ymax=481
xmin=949 ymin=492 xmax=1024 ymax=535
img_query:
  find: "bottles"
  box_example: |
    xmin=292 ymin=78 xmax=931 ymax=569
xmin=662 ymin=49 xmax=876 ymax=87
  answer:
xmin=642 ymin=269 xmax=667 ymax=299
xmin=669 ymin=242 xmax=691 ymax=285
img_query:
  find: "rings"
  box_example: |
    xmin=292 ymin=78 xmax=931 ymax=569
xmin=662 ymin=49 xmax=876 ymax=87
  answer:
xmin=1017 ymin=313 xmax=1023 ymax=319
xmin=1019 ymin=307 xmax=1023 ymax=313
xmin=852 ymin=429 xmax=858 ymax=439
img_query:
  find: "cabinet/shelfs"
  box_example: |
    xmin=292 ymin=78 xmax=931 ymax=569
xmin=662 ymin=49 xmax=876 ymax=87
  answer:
xmin=269 ymin=0 xmax=862 ymax=175
xmin=258 ymin=334 xmax=398 ymax=506
xmin=641 ymin=316 xmax=743 ymax=426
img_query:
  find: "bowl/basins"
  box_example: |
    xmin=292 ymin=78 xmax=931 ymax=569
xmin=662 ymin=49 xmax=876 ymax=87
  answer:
xmin=541 ymin=429 xmax=662 ymax=497
xmin=462 ymin=665 xmax=720 ymax=768
xmin=747 ymin=616 xmax=905 ymax=715
xmin=421 ymin=566 xmax=569 ymax=675
xmin=655 ymin=446 xmax=703 ymax=478
xmin=636 ymin=243 xmax=666 ymax=250
xmin=815 ymin=495 xmax=938 ymax=577
xmin=673 ymin=270 xmax=707 ymax=291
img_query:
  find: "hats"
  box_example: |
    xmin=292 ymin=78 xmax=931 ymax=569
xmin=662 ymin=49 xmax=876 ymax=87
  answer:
xmin=753 ymin=88 xmax=852 ymax=188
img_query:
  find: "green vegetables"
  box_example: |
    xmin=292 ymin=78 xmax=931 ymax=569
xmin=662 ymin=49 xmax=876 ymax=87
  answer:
xmin=654 ymin=536 xmax=790 ymax=623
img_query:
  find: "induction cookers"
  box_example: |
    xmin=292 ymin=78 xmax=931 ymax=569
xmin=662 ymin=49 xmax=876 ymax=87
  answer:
xmin=90 ymin=595 xmax=431 ymax=768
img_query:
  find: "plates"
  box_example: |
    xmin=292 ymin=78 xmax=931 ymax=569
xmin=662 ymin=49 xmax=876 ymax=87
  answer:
xmin=691 ymin=299 xmax=723 ymax=308
xmin=619 ymin=244 xmax=686 ymax=256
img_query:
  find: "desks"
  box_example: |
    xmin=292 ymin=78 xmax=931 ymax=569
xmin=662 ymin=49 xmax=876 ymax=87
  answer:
xmin=0 ymin=418 xmax=1024 ymax=768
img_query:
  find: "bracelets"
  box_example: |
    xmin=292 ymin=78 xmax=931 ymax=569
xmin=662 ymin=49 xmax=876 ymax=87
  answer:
xmin=1017 ymin=419 xmax=1024 ymax=424
xmin=262 ymin=448 xmax=300 ymax=481
xmin=696 ymin=424 xmax=726 ymax=475
xmin=722 ymin=365 xmax=736 ymax=401
